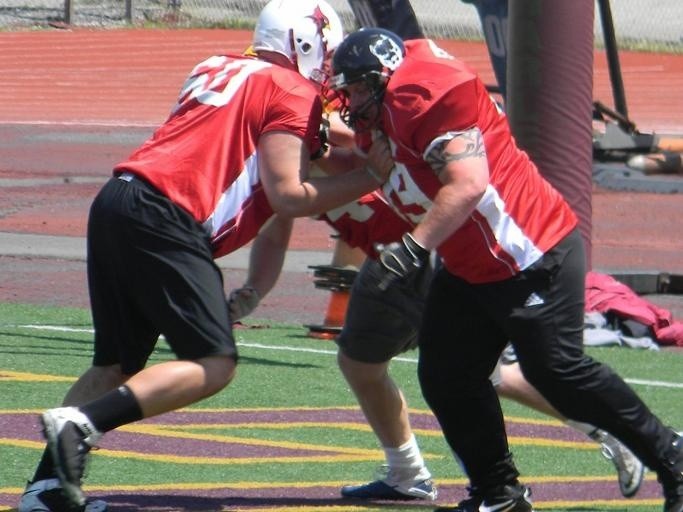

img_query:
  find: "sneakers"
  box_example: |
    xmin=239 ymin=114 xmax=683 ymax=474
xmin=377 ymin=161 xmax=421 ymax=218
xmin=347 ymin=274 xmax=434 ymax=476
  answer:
xmin=655 ymin=434 xmax=683 ymax=512
xmin=433 ymin=481 xmax=535 ymax=512
xmin=339 ymin=474 xmax=439 ymax=503
xmin=39 ymin=404 xmax=101 ymax=507
xmin=597 ymin=428 xmax=645 ymax=499
xmin=17 ymin=476 xmax=110 ymax=512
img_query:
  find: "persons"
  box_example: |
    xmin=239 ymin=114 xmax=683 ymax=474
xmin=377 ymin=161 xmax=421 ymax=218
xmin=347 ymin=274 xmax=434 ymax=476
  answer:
xmin=17 ymin=0 xmax=394 ymax=512
xmin=331 ymin=28 xmax=683 ymax=512
xmin=225 ymin=85 xmax=645 ymax=500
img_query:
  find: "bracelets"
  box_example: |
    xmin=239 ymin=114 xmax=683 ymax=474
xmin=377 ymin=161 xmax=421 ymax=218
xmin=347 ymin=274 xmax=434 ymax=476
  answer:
xmin=363 ymin=163 xmax=384 ymax=187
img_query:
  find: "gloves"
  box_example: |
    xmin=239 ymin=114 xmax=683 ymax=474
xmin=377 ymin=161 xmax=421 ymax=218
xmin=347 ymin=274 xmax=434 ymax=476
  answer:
xmin=353 ymin=230 xmax=430 ymax=308
xmin=227 ymin=284 xmax=261 ymax=322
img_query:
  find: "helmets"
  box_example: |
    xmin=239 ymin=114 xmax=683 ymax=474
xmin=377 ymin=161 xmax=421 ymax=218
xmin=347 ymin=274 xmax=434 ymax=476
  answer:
xmin=252 ymin=0 xmax=343 ymax=80
xmin=331 ymin=27 xmax=406 ymax=99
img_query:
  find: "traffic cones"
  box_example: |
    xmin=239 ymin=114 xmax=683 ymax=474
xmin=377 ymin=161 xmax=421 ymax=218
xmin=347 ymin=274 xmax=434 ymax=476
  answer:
xmin=304 ymin=228 xmax=367 ymax=338
